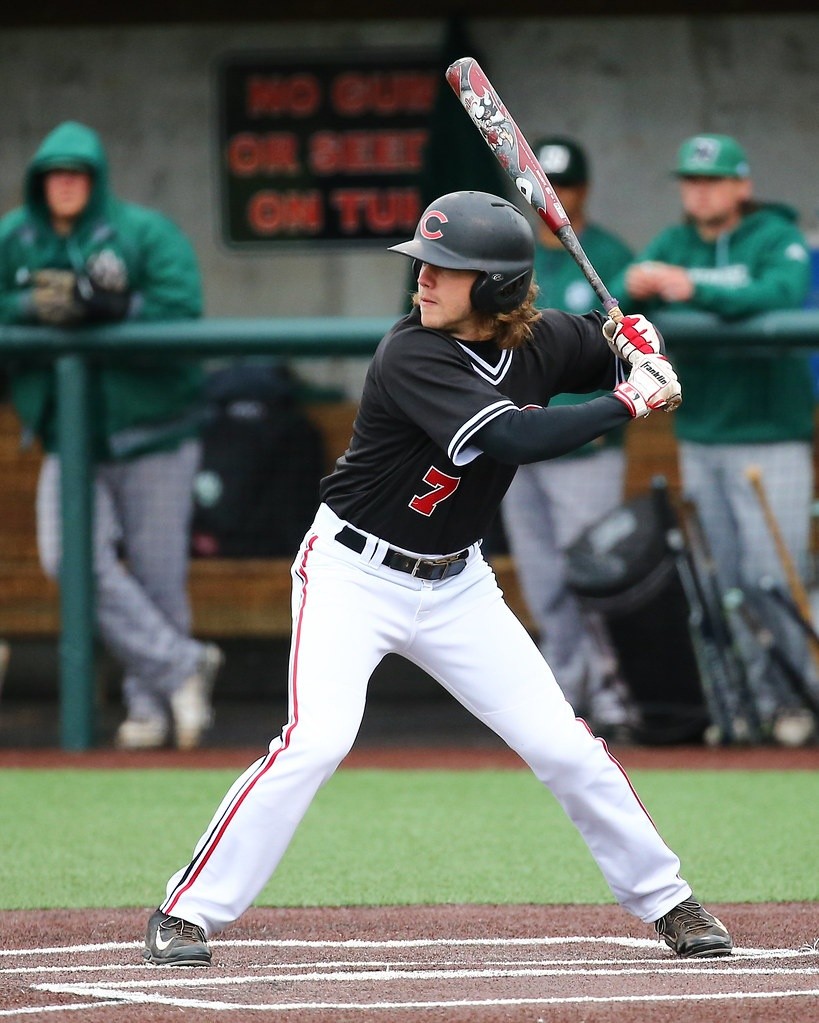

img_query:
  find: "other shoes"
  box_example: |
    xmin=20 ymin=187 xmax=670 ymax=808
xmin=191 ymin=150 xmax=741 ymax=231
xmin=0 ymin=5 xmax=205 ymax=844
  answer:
xmin=116 ymin=687 xmax=174 ymax=751
xmin=164 ymin=641 xmax=227 ymax=753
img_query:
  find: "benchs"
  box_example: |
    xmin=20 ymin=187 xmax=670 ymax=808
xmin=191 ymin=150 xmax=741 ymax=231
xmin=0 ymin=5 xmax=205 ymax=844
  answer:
xmin=0 ymin=402 xmax=533 ymax=633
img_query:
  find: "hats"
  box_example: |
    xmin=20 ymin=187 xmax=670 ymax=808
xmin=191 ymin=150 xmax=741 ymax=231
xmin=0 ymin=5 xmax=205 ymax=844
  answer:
xmin=668 ymin=132 xmax=750 ymax=184
xmin=529 ymin=136 xmax=589 ymax=190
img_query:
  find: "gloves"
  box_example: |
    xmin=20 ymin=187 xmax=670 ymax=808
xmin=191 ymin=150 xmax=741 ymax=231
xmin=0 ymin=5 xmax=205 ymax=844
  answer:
xmin=76 ymin=271 xmax=135 ymax=323
xmin=611 ymin=353 xmax=683 ymax=420
xmin=601 ymin=315 xmax=664 ymax=369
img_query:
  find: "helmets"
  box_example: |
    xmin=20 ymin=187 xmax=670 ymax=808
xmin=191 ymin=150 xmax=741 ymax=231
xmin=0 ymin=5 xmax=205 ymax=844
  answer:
xmin=385 ymin=190 xmax=536 ymax=318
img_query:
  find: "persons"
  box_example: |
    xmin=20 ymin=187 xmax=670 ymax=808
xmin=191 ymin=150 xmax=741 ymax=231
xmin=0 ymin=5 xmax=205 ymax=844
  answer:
xmin=610 ymin=132 xmax=818 ymax=747
xmin=0 ymin=120 xmax=225 ymax=753
xmin=145 ymin=189 xmax=732 ymax=958
xmin=499 ymin=133 xmax=643 ymax=739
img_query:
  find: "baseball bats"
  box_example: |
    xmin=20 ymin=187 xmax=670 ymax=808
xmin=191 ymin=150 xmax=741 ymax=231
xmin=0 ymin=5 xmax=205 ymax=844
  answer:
xmin=648 ymin=466 xmax=819 ymax=746
xmin=443 ymin=55 xmax=682 ymax=412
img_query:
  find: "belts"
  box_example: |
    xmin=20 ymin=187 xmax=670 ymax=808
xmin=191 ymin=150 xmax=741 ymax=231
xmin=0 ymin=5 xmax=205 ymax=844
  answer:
xmin=339 ymin=526 xmax=474 ymax=579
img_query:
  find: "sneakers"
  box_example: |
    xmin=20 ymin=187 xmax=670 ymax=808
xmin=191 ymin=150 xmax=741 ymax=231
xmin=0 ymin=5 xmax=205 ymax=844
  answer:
xmin=142 ymin=906 xmax=217 ymax=968
xmin=651 ymin=892 xmax=733 ymax=957
